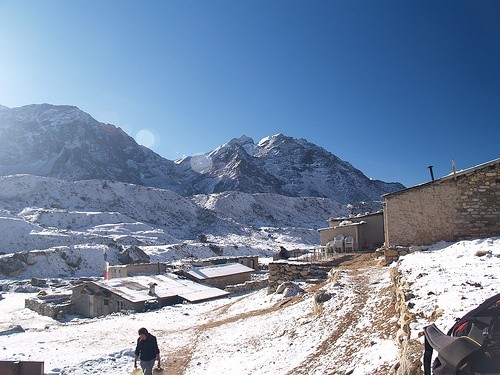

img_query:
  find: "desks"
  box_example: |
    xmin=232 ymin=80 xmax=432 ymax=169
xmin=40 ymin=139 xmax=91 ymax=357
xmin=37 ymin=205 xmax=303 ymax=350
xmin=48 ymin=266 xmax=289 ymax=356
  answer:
xmin=314 ymin=246 xmax=333 ymax=258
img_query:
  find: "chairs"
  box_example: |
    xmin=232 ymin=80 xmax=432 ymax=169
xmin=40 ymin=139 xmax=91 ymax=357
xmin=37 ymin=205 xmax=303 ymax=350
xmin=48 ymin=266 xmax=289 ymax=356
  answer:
xmin=326 ymin=234 xmax=354 ymax=253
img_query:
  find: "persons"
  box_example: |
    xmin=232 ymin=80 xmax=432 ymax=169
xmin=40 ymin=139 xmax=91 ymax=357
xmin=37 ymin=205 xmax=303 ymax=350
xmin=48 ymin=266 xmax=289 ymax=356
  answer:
xmin=133 ymin=328 xmax=162 ymax=375
xmin=279 ymin=246 xmax=290 ymax=259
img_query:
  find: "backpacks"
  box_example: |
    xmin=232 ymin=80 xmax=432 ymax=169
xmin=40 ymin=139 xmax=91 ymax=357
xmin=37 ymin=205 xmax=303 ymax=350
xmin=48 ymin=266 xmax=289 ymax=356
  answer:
xmin=424 ymin=292 xmax=499 ymax=374
